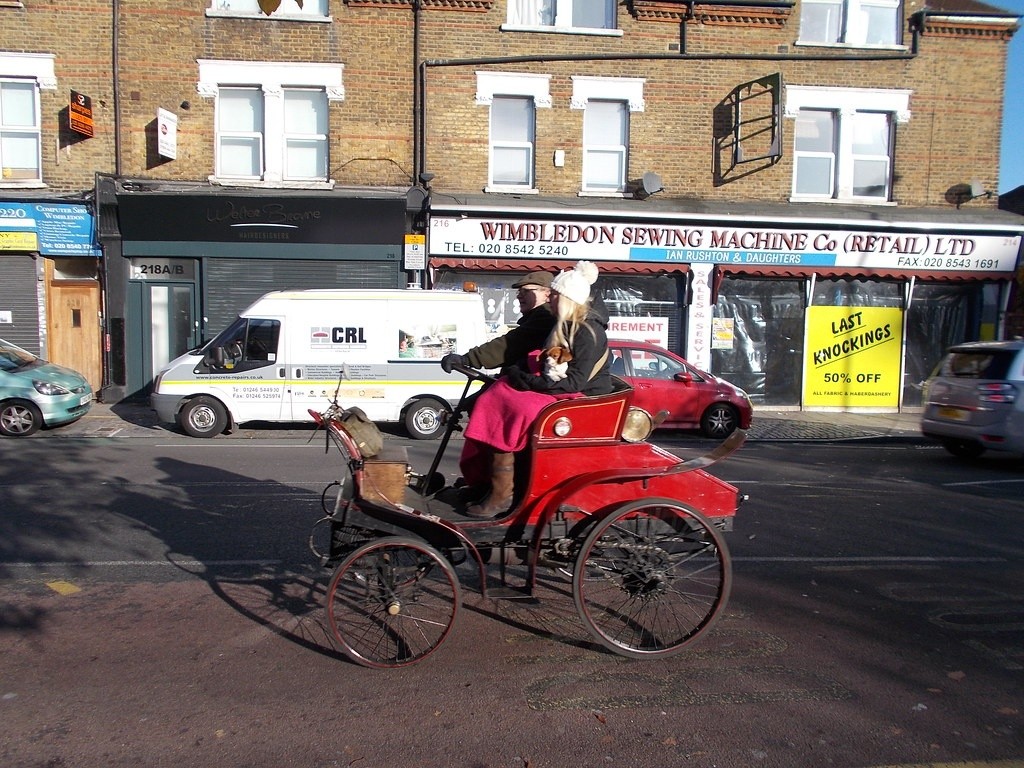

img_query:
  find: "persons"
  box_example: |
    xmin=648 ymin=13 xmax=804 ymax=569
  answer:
xmin=443 ymin=260 xmax=614 ymax=520
xmin=440 ymin=270 xmax=561 ymax=384
xmin=399 ymin=339 xmax=415 ymax=358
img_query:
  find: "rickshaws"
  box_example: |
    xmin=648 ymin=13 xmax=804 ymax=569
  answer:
xmin=299 ymin=358 xmax=745 ymax=670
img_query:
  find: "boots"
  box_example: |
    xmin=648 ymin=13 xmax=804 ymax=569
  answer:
xmin=465 ymin=452 xmax=513 ymax=518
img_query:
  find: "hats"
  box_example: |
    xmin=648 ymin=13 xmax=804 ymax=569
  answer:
xmin=552 ymin=260 xmax=599 ymax=306
xmin=511 ymin=270 xmax=554 ymax=290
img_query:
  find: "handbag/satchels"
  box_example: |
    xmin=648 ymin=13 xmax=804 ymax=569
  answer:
xmin=334 ymin=406 xmax=383 ymax=458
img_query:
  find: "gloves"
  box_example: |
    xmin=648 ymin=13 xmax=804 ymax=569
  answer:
xmin=442 ymin=353 xmax=466 ymax=373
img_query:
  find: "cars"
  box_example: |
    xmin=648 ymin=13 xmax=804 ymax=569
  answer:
xmin=1 ymin=337 xmax=92 ymax=435
xmin=921 ymin=342 xmax=1023 ymax=461
xmin=604 ymin=334 xmax=754 ymax=438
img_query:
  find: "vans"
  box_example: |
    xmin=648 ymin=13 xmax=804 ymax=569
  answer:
xmin=148 ymin=288 xmax=487 ymax=442
